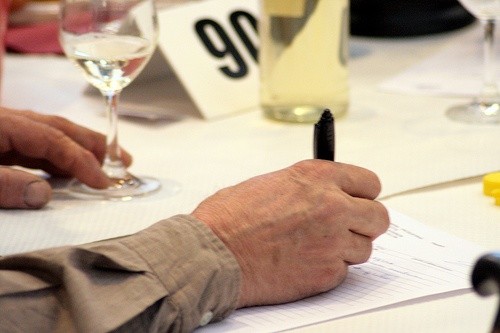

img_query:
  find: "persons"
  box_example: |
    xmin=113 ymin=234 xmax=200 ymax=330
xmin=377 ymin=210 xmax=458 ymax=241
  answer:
xmin=0 ymin=107 xmax=389 ymax=333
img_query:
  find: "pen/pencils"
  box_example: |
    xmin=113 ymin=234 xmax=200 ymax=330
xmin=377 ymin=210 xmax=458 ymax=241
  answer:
xmin=314 ymin=108 xmax=334 ymax=161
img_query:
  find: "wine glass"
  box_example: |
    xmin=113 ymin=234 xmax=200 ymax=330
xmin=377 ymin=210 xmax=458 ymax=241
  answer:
xmin=445 ymin=0 xmax=500 ymax=125
xmin=58 ymin=0 xmax=162 ymax=200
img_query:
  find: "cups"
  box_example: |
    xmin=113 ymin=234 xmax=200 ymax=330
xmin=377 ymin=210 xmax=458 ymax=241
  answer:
xmin=258 ymin=0 xmax=350 ymax=124
